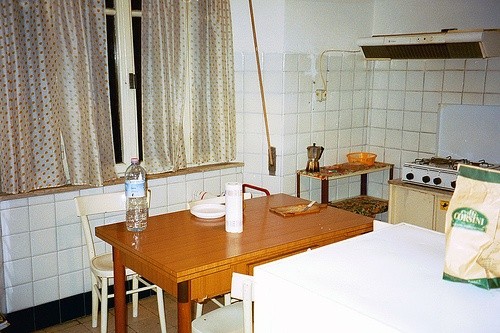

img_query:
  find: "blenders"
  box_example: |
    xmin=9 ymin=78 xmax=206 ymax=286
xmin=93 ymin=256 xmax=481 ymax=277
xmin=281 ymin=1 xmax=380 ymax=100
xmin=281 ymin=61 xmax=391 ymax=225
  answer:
xmin=306 ymin=142 xmax=324 ymax=172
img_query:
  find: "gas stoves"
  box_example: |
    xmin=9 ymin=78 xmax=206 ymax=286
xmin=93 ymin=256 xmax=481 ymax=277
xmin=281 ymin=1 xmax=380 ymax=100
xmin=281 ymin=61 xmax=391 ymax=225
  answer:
xmin=401 ymin=156 xmax=500 ymax=192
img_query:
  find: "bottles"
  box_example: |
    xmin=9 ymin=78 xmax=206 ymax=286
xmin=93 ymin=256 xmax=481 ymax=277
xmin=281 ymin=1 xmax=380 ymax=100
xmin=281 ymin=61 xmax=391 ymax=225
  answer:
xmin=125 ymin=158 xmax=148 ymax=232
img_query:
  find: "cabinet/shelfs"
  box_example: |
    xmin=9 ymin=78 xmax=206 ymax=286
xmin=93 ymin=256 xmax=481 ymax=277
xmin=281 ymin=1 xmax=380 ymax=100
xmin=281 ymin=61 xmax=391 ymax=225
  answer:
xmin=388 ymin=184 xmax=453 ymax=233
xmin=298 ymin=162 xmax=394 ymax=218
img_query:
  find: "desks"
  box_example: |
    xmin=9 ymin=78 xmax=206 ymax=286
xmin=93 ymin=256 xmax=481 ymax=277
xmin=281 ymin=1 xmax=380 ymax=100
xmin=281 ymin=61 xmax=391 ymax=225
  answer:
xmin=94 ymin=193 xmax=374 ymax=332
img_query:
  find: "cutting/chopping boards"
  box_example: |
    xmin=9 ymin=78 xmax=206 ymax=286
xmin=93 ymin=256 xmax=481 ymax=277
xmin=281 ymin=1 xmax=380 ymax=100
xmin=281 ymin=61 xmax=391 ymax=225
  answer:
xmin=269 ymin=202 xmax=327 ymax=219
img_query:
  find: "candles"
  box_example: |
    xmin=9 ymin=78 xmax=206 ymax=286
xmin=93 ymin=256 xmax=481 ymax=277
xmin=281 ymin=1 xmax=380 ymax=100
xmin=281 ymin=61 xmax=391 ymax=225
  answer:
xmin=226 ymin=182 xmax=244 ymax=234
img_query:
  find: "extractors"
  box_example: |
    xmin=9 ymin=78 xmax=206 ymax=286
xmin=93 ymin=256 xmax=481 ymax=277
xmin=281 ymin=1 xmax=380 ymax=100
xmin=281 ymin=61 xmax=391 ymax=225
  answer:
xmin=356 ymin=29 xmax=500 ymax=61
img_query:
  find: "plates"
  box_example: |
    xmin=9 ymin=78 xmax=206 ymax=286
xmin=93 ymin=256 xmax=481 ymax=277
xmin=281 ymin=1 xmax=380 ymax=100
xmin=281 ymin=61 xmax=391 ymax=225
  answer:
xmin=189 ymin=204 xmax=226 ymax=219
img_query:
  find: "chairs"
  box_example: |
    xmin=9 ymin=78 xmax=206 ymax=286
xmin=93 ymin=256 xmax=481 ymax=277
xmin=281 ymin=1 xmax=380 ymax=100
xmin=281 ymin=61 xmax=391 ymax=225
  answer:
xmin=74 ymin=189 xmax=167 ymax=333
xmin=187 ymin=193 xmax=252 ymax=319
xmin=191 ymin=248 xmax=311 ymax=333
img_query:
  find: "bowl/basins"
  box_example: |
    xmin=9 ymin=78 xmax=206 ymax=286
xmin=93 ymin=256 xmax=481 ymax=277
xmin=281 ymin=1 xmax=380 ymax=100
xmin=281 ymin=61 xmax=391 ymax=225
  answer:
xmin=347 ymin=152 xmax=377 ymax=168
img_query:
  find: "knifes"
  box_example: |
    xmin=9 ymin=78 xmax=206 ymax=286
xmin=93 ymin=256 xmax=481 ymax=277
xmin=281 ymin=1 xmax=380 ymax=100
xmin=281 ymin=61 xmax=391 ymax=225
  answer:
xmin=303 ymin=200 xmax=316 ymax=212
